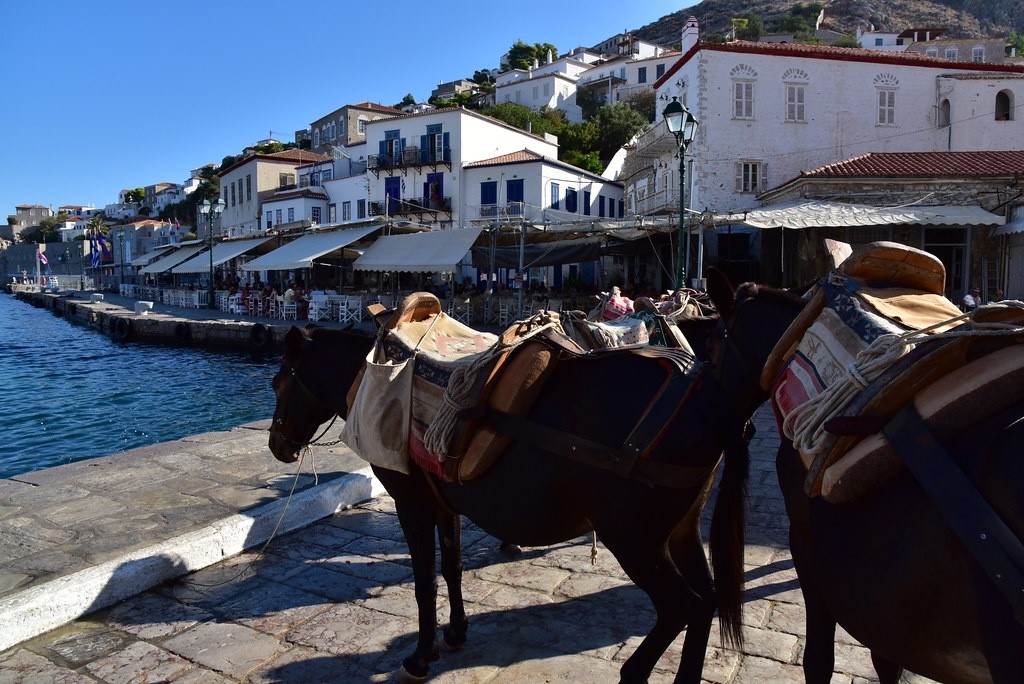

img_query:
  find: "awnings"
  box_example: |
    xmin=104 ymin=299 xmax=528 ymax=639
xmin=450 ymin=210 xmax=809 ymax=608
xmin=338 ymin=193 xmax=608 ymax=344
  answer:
xmin=131 ymin=248 xmax=170 ymax=266
xmin=138 ymin=246 xmax=207 ymax=275
xmin=745 ymin=198 xmax=1024 ymax=237
xmin=237 ymin=223 xmax=386 ymax=271
xmin=352 ymin=225 xmax=484 ymax=271
xmin=171 ymin=236 xmax=275 ymax=273
xmin=428 ymin=278 xmax=548 ymax=297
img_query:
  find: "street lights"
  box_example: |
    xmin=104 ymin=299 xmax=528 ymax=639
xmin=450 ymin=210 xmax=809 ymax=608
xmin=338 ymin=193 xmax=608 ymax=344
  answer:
xmin=77 ymin=242 xmax=87 ymax=292
xmin=117 ymin=225 xmax=126 ymax=297
xmin=65 ymin=247 xmax=72 ymax=285
xmin=661 ymin=95 xmax=699 ymax=292
xmin=198 ymin=196 xmax=227 ymax=310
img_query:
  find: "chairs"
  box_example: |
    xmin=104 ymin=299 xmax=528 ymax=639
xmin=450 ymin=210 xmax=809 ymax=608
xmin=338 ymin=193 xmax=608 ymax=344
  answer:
xmin=371 ymin=203 xmax=382 ymax=216
xmin=120 ymin=284 xmax=208 ymax=309
xmin=214 ymin=290 xmax=564 ymax=327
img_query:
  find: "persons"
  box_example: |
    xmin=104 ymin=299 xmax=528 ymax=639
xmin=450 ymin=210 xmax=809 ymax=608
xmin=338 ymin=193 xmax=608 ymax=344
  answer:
xmin=959 ymin=287 xmax=1004 ymax=312
xmin=189 ymin=274 xmax=331 ymax=319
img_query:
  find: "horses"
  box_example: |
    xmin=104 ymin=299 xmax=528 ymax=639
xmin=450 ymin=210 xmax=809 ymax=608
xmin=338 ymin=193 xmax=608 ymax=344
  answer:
xmin=267 ymin=265 xmax=1024 ymax=684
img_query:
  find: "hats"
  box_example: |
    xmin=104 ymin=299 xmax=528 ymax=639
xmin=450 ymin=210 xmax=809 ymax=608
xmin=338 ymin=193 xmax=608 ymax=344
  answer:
xmin=973 ymin=288 xmax=981 ymax=292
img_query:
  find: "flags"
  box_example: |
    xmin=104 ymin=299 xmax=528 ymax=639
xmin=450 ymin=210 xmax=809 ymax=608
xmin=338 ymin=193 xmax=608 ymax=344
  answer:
xmin=37 ymin=250 xmax=48 ymax=264
xmin=175 ymin=218 xmax=180 ymax=232
xmin=98 ymin=231 xmax=111 ymax=261
xmin=169 ymin=219 xmax=172 ymax=230
xmin=89 ymin=235 xmax=100 ymax=268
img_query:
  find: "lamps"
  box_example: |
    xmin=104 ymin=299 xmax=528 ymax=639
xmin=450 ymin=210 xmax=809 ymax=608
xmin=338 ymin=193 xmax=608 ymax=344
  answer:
xmin=673 ymin=151 xmax=680 ymax=158
xmin=659 ymin=92 xmax=668 ymax=100
xmin=675 ymin=78 xmax=686 ymax=88
xmin=658 ymin=160 xmax=667 ymax=169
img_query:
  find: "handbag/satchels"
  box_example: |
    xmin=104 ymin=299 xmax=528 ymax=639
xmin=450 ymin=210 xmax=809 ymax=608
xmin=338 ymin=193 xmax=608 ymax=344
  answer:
xmin=339 ymin=343 xmax=415 ymax=475
